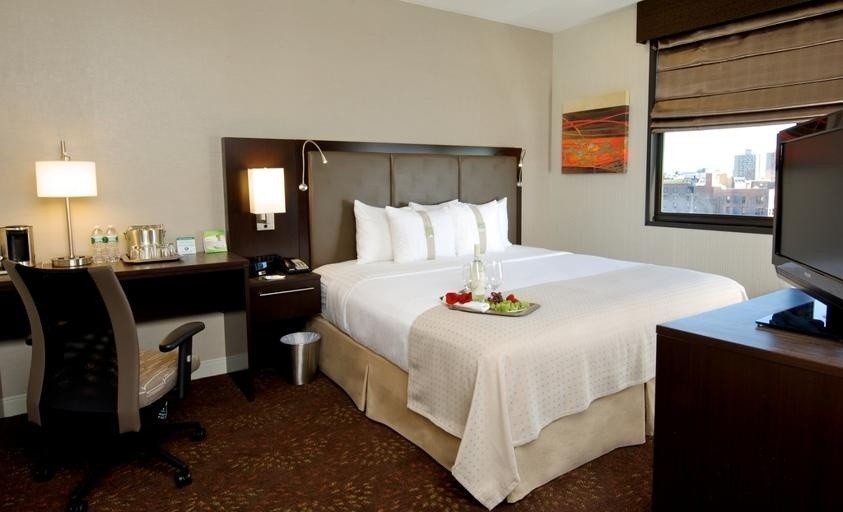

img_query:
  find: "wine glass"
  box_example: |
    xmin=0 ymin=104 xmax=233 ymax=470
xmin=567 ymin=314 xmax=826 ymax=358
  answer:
xmin=465 ymin=258 xmax=503 ymax=302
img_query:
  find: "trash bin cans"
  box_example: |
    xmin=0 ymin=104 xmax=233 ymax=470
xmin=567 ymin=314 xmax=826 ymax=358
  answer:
xmin=279 ymin=331 xmax=322 ymax=386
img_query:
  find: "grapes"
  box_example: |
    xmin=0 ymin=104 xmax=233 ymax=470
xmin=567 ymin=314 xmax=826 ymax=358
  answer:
xmin=487 ymin=292 xmax=529 ymax=312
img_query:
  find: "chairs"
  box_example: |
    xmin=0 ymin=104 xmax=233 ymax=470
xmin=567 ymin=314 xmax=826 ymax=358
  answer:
xmin=2 ymin=259 xmax=207 ymax=511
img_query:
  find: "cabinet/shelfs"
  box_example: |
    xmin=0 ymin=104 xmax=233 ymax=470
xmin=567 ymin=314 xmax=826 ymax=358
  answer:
xmin=651 ymin=291 xmax=842 ymax=511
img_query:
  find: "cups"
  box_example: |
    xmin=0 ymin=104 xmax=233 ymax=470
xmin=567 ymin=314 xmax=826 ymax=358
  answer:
xmin=128 ymin=242 xmax=177 ymax=261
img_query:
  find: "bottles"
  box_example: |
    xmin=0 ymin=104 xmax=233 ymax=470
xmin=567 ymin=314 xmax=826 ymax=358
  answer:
xmin=90 ymin=225 xmax=106 ymax=264
xmin=104 ymin=225 xmax=122 ymax=262
xmin=474 ymin=244 xmax=482 ymax=263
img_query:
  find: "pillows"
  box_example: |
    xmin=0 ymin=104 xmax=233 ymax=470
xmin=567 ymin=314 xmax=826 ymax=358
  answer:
xmin=347 ymin=194 xmax=514 ymax=263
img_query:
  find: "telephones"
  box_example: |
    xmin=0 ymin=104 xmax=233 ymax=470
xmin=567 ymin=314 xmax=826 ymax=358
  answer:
xmin=279 ymin=257 xmax=311 ymax=274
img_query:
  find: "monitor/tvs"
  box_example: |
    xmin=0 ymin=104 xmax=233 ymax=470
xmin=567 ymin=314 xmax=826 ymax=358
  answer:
xmin=772 ymin=109 xmax=843 ymax=339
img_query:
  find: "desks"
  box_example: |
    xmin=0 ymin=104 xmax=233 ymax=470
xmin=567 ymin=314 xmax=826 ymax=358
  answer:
xmin=0 ymin=245 xmax=256 ymax=404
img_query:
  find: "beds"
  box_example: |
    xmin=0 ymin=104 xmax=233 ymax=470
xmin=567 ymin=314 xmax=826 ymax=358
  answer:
xmin=305 ymin=147 xmax=747 ymax=512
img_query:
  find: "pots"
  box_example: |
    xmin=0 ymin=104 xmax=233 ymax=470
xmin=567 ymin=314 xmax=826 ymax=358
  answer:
xmin=124 ymin=223 xmax=165 ymax=257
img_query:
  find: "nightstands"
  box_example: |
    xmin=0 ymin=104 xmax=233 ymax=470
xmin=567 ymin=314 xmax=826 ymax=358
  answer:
xmin=248 ymin=269 xmax=321 ymax=322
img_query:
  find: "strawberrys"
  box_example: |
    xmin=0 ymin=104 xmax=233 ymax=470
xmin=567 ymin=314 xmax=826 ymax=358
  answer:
xmin=446 ymin=292 xmax=472 ymax=305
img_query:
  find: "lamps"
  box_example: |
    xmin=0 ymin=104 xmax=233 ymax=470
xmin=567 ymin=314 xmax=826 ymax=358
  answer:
xmin=246 ymin=164 xmax=287 ymax=232
xmin=514 ymin=148 xmax=529 ymax=188
xmin=296 ymin=140 xmax=326 ymax=192
xmin=31 ymin=140 xmax=99 ymax=268
